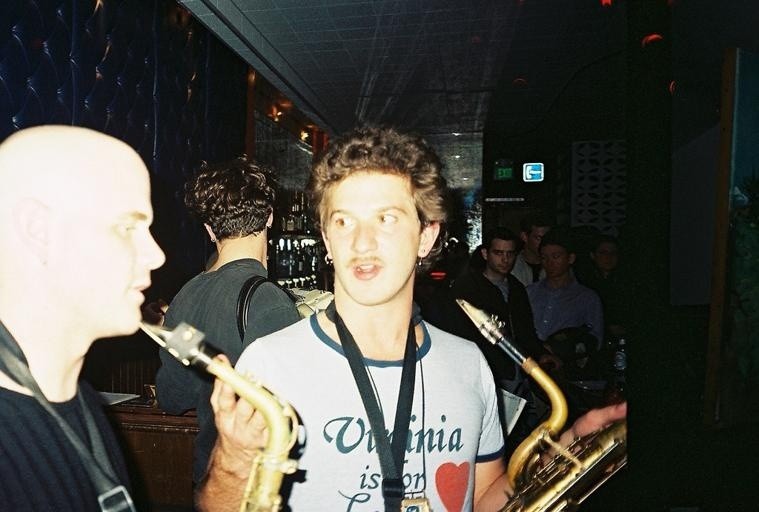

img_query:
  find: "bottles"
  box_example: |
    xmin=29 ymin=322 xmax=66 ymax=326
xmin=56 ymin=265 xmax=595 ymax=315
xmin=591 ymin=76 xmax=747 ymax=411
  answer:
xmin=276 ymin=239 xmax=319 ymax=277
xmin=613 ymin=336 xmax=626 ymax=376
xmin=281 ymin=187 xmax=309 ymax=232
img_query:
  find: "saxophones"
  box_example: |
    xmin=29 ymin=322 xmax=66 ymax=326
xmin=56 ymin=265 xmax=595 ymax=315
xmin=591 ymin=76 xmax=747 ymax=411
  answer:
xmin=140 ymin=320 xmax=299 ymax=512
xmin=456 ymin=299 xmax=629 ymax=512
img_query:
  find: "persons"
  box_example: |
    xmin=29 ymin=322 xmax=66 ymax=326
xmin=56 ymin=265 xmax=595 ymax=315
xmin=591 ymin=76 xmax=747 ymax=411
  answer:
xmin=141 ymin=156 xmax=301 ymax=366
xmin=236 ymin=127 xmax=629 ymax=511
xmin=0 ymin=124 xmax=269 ymax=512
xmin=449 ymin=212 xmax=630 ymax=445
